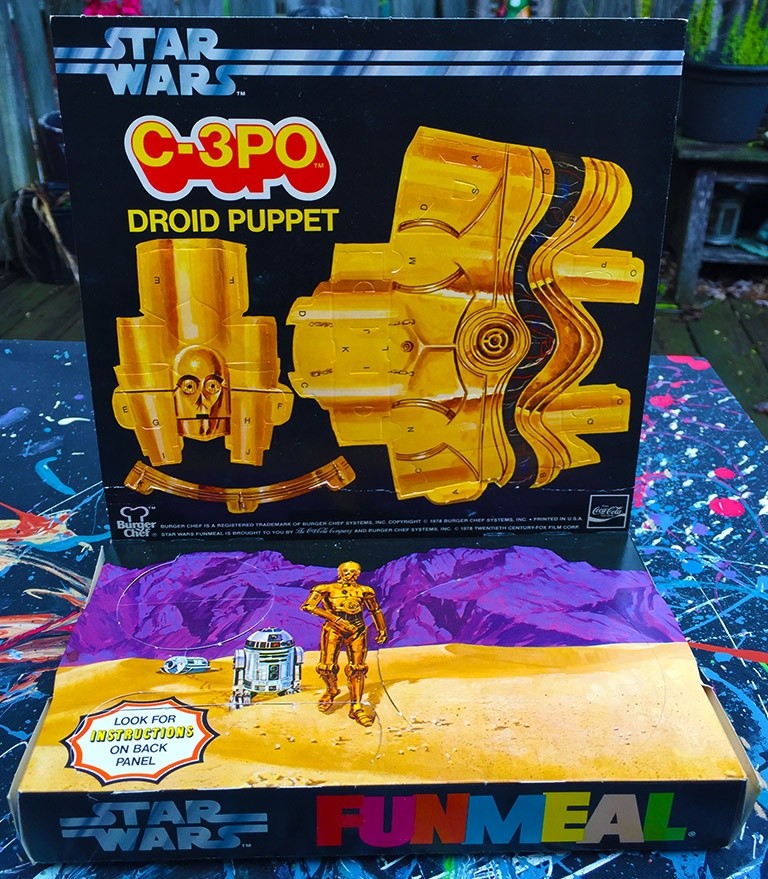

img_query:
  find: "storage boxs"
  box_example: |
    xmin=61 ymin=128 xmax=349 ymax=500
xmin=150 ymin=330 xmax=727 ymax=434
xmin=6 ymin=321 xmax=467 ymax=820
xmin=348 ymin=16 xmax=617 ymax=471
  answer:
xmin=5 ymin=529 xmax=762 ymax=866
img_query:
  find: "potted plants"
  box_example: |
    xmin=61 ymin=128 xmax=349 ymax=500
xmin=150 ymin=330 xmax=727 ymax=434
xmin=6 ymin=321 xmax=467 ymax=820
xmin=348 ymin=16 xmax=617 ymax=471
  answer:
xmin=641 ymin=0 xmax=768 ymax=143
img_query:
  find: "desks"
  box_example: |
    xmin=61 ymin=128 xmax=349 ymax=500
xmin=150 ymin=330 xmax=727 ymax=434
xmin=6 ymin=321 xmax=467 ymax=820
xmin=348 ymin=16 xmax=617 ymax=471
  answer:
xmin=0 ymin=337 xmax=768 ymax=879
xmin=674 ymin=135 xmax=768 ymax=308
